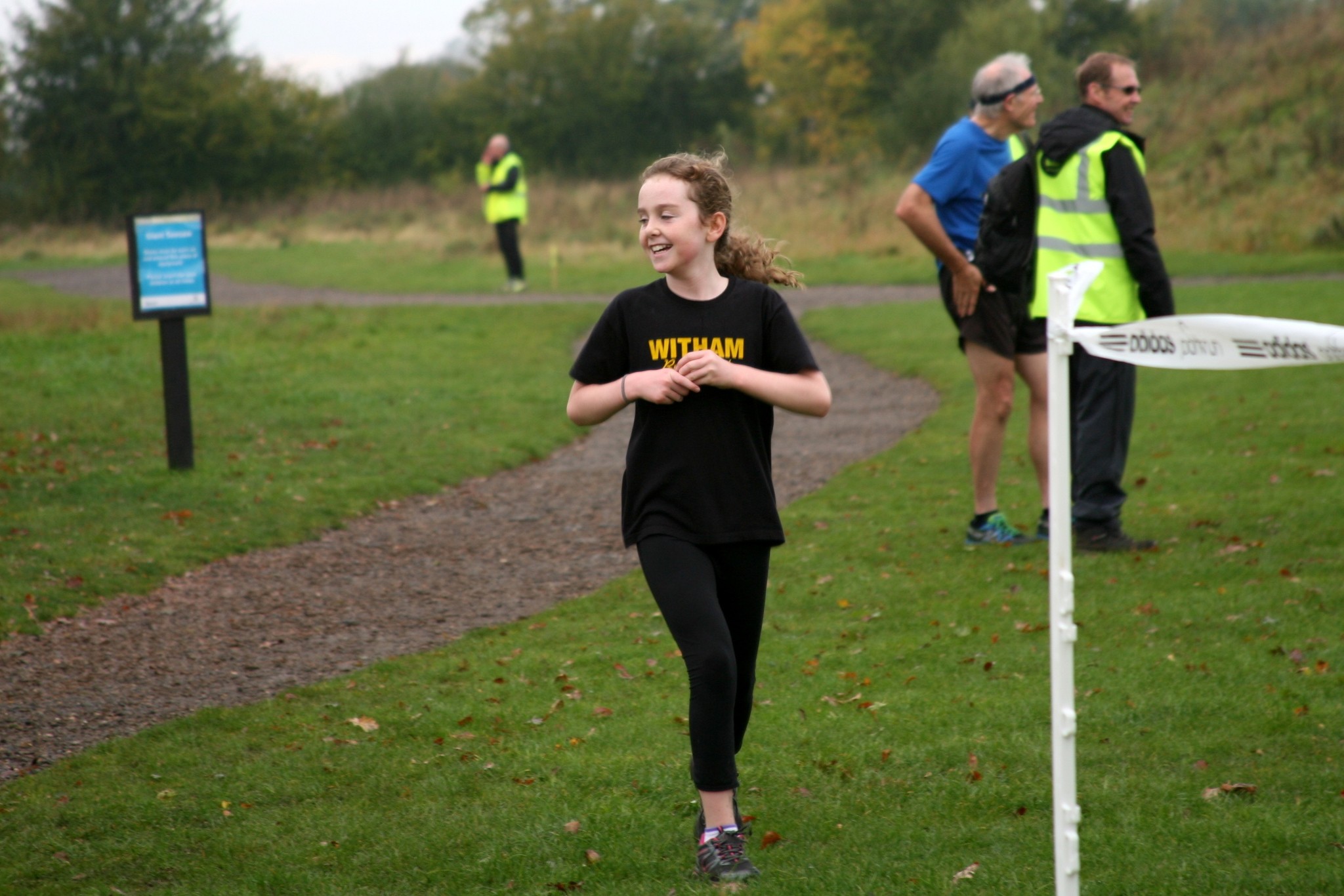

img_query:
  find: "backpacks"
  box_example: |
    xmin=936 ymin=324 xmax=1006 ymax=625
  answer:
xmin=974 ymin=140 xmax=1043 ymax=303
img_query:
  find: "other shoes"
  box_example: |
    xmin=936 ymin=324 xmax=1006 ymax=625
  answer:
xmin=1074 ymin=530 xmax=1159 ymax=553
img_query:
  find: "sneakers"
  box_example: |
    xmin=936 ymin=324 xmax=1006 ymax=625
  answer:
xmin=697 ymin=825 xmax=761 ymax=884
xmin=694 ymin=800 xmax=744 ymax=847
xmin=1036 ymin=518 xmax=1049 ymax=539
xmin=964 ymin=512 xmax=1032 ymax=551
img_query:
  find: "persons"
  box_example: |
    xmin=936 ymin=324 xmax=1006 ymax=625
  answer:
xmin=565 ymin=150 xmax=832 ymax=880
xmin=894 ymin=51 xmax=1049 ymax=548
xmin=474 ymin=132 xmax=529 ymax=293
xmin=1023 ymin=51 xmax=1176 ymax=552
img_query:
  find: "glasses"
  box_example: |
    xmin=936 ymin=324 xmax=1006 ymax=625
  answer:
xmin=1105 ymin=83 xmax=1143 ymax=96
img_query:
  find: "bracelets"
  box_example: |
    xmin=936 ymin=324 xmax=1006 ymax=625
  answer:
xmin=621 ymin=373 xmax=634 ymax=405
